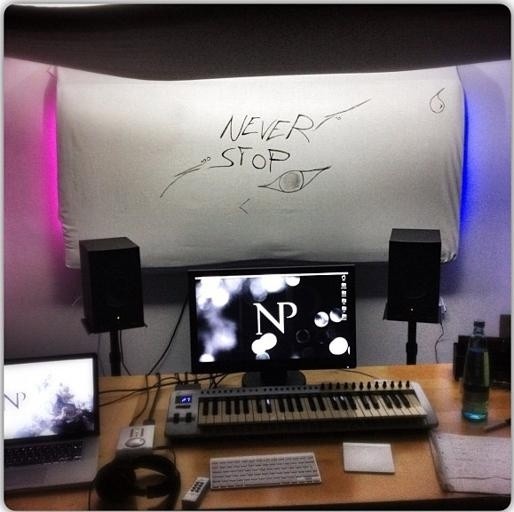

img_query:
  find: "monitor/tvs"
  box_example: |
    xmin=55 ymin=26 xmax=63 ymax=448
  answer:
xmin=186 ymin=264 xmax=357 ymax=388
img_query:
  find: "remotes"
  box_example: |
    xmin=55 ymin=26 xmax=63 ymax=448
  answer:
xmin=181 ymin=476 xmax=210 ymax=507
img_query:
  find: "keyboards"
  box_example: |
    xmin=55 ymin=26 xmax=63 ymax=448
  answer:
xmin=209 ymin=452 xmax=322 ymax=490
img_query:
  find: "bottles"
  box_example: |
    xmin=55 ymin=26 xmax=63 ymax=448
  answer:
xmin=461 ymin=320 xmax=491 ymax=423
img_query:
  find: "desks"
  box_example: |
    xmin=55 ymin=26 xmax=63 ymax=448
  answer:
xmin=4 ymin=363 xmax=510 ymax=510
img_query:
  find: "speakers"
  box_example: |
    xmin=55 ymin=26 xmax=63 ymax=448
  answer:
xmin=383 ymin=228 xmax=442 ymax=324
xmin=78 ymin=236 xmax=145 ymax=336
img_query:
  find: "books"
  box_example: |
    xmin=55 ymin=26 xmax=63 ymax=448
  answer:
xmin=428 ymin=428 xmax=512 ymax=498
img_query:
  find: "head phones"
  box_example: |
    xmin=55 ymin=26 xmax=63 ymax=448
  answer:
xmin=92 ymin=448 xmax=181 ymax=511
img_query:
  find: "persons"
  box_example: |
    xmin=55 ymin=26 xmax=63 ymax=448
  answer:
xmin=12 ymin=379 xmax=94 ymax=438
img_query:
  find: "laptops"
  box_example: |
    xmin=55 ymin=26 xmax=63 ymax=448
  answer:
xmin=4 ymin=352 xmax=101 ymax=495
